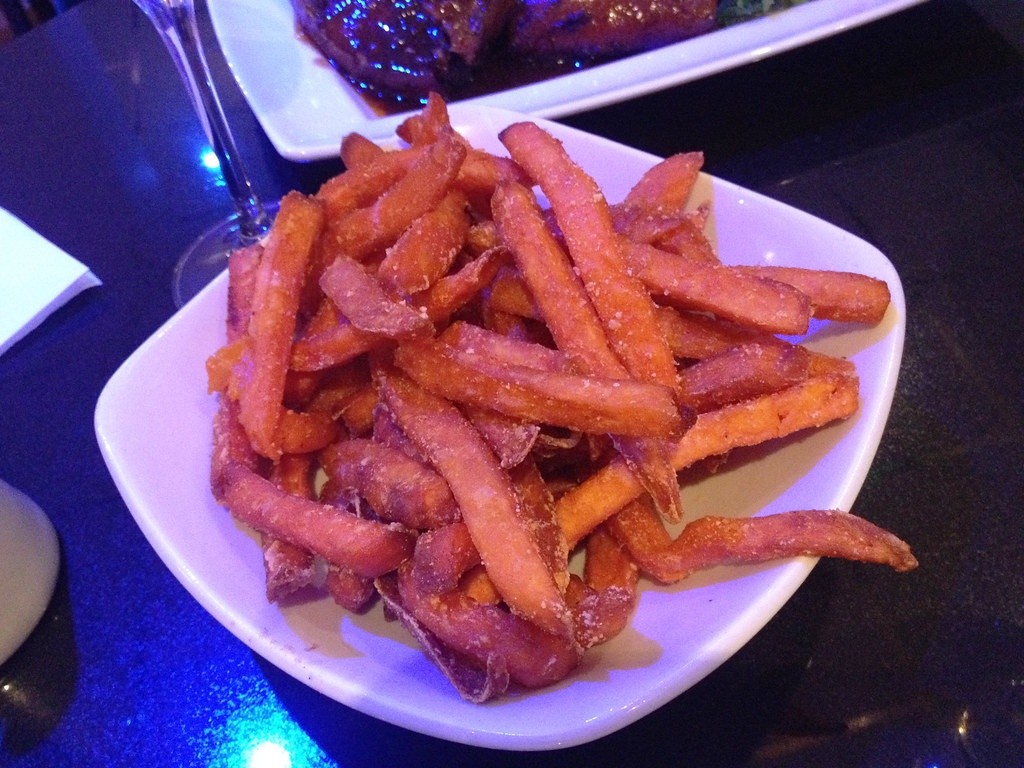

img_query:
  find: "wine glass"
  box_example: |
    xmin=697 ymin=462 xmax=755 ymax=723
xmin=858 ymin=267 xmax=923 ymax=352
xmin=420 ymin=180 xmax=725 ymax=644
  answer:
xmin=132 ymin=0 xmax=284 ymax=314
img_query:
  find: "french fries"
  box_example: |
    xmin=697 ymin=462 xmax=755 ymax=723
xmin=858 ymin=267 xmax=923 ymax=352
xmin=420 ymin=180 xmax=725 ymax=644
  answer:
xmin=209 ymin=98 xmax=940 ymax=683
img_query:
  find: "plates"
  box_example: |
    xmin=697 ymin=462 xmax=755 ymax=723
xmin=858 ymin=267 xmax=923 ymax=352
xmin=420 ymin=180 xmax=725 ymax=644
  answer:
xmin=87 ymin=98 xmax=907 ymax=756
xmin=203 ymin=0 xmax=910 ymax=158
